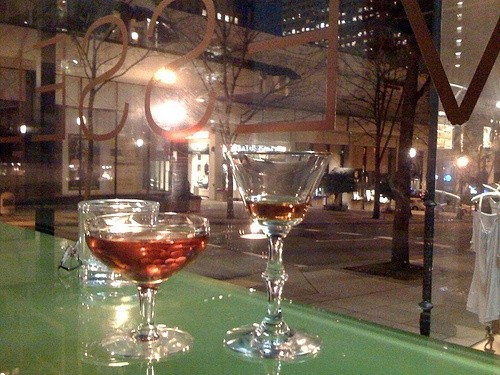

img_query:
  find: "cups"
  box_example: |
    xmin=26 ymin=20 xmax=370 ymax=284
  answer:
xmin=77 ymin=198 xmax=160 ymax=281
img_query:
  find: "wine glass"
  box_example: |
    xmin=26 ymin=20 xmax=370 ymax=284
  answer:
xmin=83 ymin=212 xmax=210 ymax=362
xmin=222 ymin=150 xmax=333 ymax=360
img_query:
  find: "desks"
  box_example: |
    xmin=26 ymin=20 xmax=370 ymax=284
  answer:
xmin=0 ymin=222 xmax=500 ymax=375
